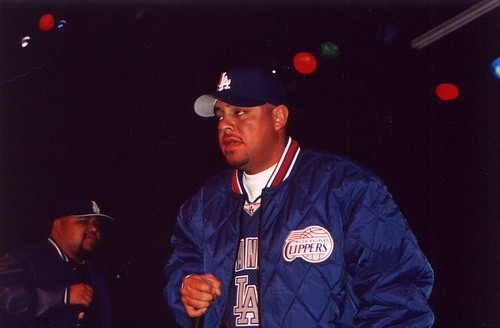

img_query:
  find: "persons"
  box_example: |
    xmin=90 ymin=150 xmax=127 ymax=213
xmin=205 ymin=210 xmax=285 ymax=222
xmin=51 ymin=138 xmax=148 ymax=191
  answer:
xmin=0 ymin=200 xmax=115 ymax=328
xmin=160 ymin=67 xmax=436 ymax=328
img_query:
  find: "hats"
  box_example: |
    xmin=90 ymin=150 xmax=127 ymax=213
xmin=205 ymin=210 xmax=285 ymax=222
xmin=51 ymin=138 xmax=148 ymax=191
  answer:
xmin=51 ymin=194 xmax=115 ymax=222
xmin=194 ymin=68 xmax=287 ymax=118
